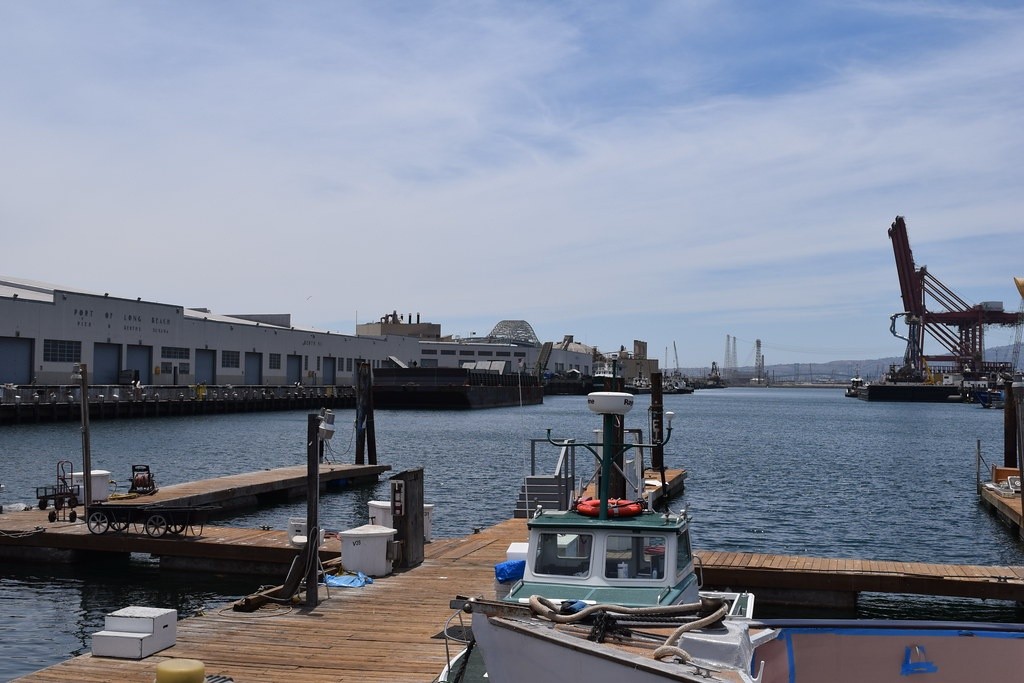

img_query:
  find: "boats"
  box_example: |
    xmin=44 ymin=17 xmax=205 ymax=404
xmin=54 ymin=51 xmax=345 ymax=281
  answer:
xmin=437 ymin=591 xmax=1024 ymax=683
xmin=844 ymin=363 xmax=872 ymax=397
xmin=593 ymin=332 xmax=695 ymax=394
xmin=974 ymin=387 xmax=1005 ymax=410
xmin=499 ymin=386 xmax=757 ymax=619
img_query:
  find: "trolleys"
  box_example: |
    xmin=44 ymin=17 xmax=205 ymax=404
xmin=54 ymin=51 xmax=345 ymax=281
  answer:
xmin=31 ymin=460 xmax=80 ymax=523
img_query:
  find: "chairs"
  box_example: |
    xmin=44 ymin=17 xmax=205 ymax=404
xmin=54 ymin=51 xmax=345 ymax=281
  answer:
xmin=126 ymin=464 xmax=157 ymax=496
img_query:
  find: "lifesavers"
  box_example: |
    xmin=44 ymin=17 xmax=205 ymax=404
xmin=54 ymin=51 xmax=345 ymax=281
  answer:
xmin=577 ymin=497 xmax=641 ymax=517
